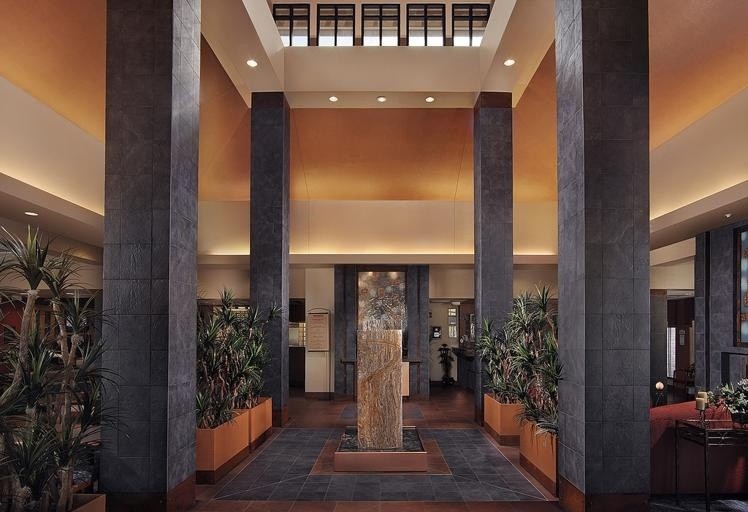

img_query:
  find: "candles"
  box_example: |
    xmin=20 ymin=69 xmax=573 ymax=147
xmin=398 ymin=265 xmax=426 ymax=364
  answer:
xmin=695 ymin=390 xmax=707 ymax=410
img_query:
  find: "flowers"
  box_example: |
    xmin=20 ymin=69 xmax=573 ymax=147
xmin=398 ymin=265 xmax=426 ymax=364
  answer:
xmin=706 ymin=376 xmax=748 ymax=414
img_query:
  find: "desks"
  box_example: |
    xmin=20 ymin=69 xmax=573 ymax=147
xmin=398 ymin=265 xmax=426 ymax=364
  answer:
xmin=673 ymin=418 xmax=748 ymax=512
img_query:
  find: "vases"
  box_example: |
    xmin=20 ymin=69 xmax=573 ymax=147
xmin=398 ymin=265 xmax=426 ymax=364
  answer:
xmin=731 ymin=413 xmax=747 ymax=424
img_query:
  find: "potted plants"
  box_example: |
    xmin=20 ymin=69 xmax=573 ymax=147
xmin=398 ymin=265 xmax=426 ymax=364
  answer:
xmin=182 ymin=285 xmax=289 ymax=487
xmin=473 ymin=277 xmax=563 ymax=497
